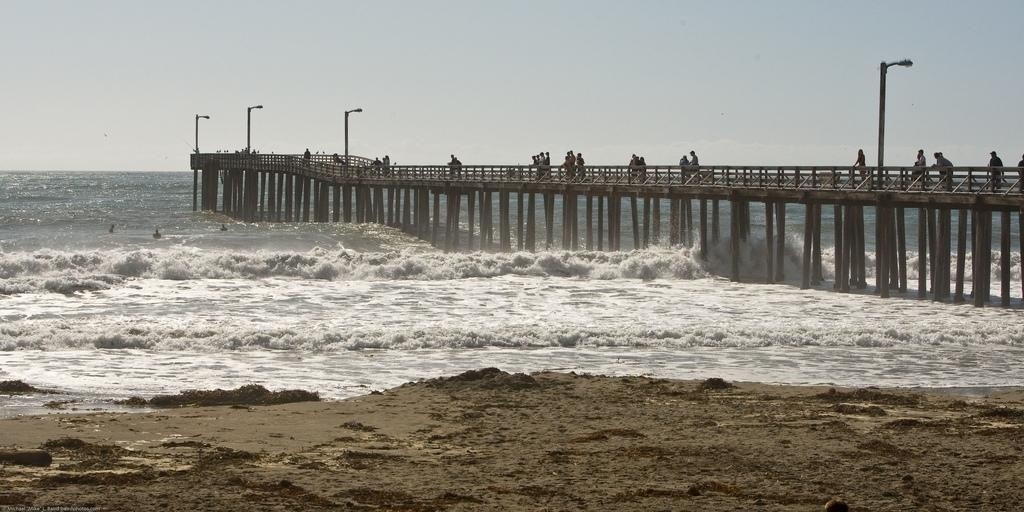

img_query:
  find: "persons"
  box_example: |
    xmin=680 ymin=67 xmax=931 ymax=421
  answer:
xmin=382 ymin=158 xmax=387 ymax=177
xmin=530 ymin=149 xmax=587 ymax=182
xmin=639 ymin=157 xmax=648 ymax=183
xmin=333 ymin=152 xmax=345 ymax=165
xmin=989 ymin=150 xmax=1005 ymax=191
xmin=384 ymin=155 xmax=390 ymax=176
xmin=852 ymin=149 xmax=867 ymax=186
xmin=1017 ymin=154 xmax=1024 ymax=192
xmin=690 ymin=150 xmax=701 ymax=184
xmin=304 ymin=148 xmax=311 ymax=165
xmin=448 ymin=154 xmax=456 ymax=176
xmin=679 ymin=155 xmax=693 ymax=184
xmin=931 ymin=152 xmax=954 ymax=182
xmin=371 ymin=157 xmax=382 ymax=177
xmin=915 ymin=149 xmax=926 ymax=189
xmin=455 ymin=157 xmax=463 ymax=175
xmin=628 ymin=154 xmax=640 ymax=181
xmin=938 ymin=152 xmax=943 ymax=181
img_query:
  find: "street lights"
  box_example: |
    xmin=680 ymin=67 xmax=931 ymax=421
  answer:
xmin=875 ymin=59 xmax=915 ymax=189
xmin=341 ymin=110 xmax=365 ymax=165
xmin=194 ymin=113 xmax=209 ymax=153
xmin=244 ymin=104 xmax=263 ymax=156
